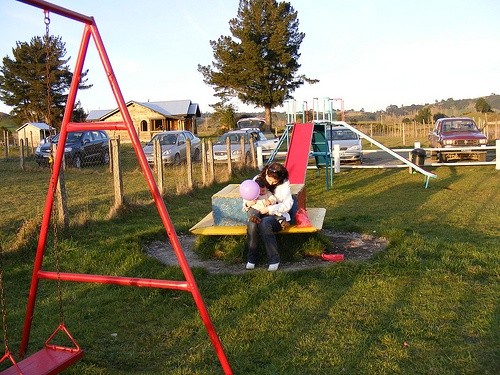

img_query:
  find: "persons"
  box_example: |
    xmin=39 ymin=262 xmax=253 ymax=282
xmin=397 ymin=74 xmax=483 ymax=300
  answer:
xmin=246 ymin=177 xmax=288 ymax=228
xmin=242 ymin=163 xmax=295 ymax=270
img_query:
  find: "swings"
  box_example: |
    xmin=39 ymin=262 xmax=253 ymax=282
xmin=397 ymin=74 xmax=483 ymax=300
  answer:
xmin=0 ymin=7 xmax=88 ymax=375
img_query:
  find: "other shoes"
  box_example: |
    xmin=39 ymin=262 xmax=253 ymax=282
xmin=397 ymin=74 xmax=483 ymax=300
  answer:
xmin=267 ymin=261 xmax=280 ymax=272
xmin=250 ymin=215 xmax=261 ymax=224
xmin=281 ymin=220 xmax=290 ymax=231
xmin=246 ymin=262 xmax=255 ymax=270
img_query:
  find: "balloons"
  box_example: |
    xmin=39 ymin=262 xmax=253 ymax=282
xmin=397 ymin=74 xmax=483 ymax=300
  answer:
xmin=240 ymin=179 xmax=260 ymax=201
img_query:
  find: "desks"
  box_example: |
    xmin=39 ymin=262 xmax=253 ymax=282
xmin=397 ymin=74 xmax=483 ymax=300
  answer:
xmin=212 ymin=182 xmax=306 ymax=226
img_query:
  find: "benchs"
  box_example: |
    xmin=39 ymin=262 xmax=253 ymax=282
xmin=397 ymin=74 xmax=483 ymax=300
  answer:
xmin=187 ymin=206 xmax=326 ymax=236
xmin=440 ymin=152 xmax=487 ymax=162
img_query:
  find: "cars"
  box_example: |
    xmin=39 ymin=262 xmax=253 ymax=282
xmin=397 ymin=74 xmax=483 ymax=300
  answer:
xmin=327 ymin=127 xmax=363 ymax=164
xmin=207 ymin=129 xmax=280 ymax=164
xmin=35 ymin=130 xmax=112 ymax=169
xmin=429 ymin=117 xmax=487 ymax=162
xmin=141 ymin=130 xmax=202 ymax=166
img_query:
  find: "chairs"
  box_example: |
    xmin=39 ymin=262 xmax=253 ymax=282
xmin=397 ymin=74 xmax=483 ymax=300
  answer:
xmin=460 ymin=124 xmax=472 ymax=130
xmin=444 ymin=124 xmax=450 ymax=131
xmin=92 ymin=134 xmax=97 ymax=140
xmin=230 ymin=135 xmax=237 ymax=141
xmin=251 ymin=121 xmax=259 ymax=126
xmin=163 ymin=136 xmax=169 ymax=142
xmin=68 ymin=134 xmax=74 ymax=140
xmin=169 ymin=138 xmax=176 ymax=144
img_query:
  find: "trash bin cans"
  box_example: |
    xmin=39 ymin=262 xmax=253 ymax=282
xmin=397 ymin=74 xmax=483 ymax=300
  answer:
xmin=412 ymin=148 xmax=427 ymax=169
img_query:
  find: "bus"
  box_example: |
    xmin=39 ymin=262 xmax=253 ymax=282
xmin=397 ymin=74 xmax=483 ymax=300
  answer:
xmin=236 ymin=117 xmax=266 ymax=132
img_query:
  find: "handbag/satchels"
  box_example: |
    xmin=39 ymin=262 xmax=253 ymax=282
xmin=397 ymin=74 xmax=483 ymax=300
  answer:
xmin=295 ymin=208 xmax=313 ymax=228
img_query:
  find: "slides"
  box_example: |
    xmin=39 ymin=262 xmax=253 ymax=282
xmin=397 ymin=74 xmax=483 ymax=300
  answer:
xmin=311 ymin=123 xmax=331 ymax=168
xmin=340 ymin=121 xmax=438 ymax=189
xmin=284 ymin=123 xmax=314 ymax=184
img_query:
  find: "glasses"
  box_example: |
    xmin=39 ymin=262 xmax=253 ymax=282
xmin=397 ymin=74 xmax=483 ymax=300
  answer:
xmin=268 ymin=168 xmax=285 ymax=180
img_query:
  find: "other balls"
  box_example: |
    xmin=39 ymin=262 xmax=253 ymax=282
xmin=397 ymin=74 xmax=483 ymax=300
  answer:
xmin=240 ymin=180 xmax=260 ymax=200
